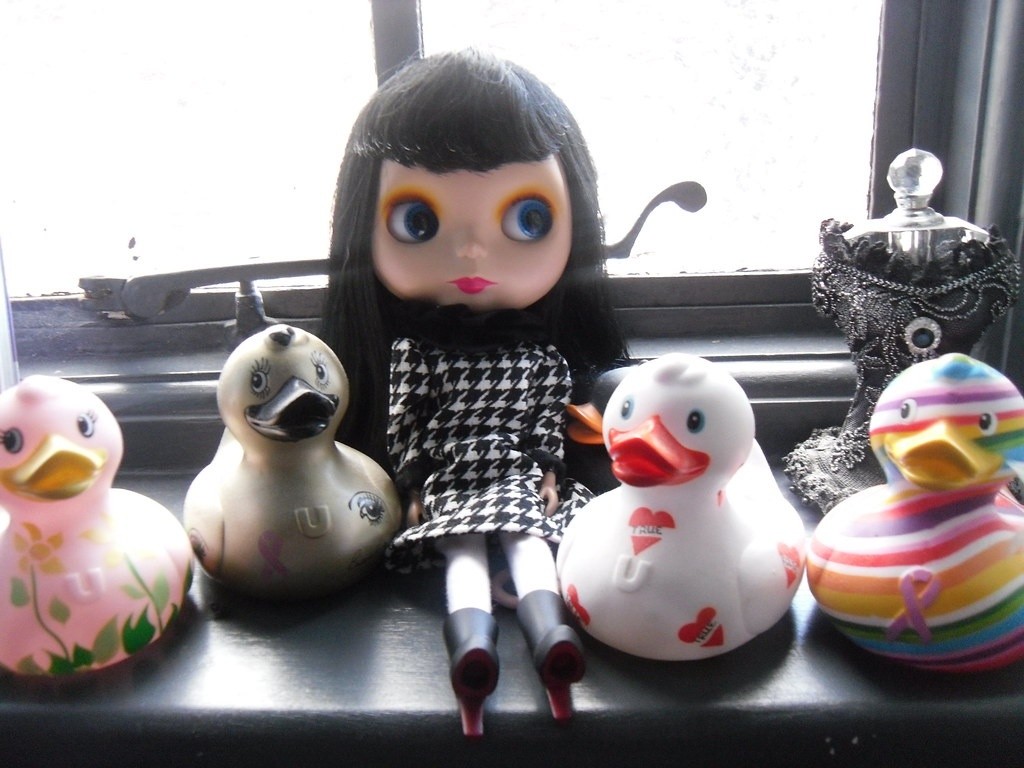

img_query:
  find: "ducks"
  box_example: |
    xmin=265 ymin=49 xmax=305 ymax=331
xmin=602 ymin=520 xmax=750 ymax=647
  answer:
xmin=806 ymin=352 xmax=1024 ymax=673
xmin=555 ymin=353 xmax=805 ymax=663
xmin=0 ymin=373 xmax=194 ymax=681
xmin=178 ymin=322 xmax=404 ymax=606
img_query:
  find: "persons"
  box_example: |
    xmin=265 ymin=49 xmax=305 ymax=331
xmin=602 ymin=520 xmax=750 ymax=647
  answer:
xmin=316 ymin=48 xmax=626 ymax=743
